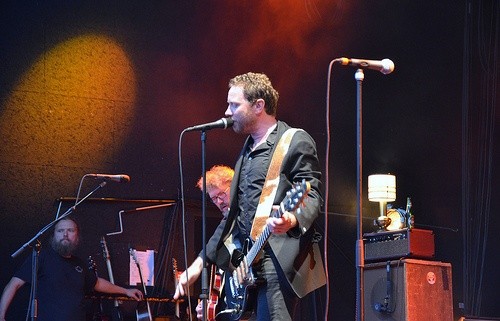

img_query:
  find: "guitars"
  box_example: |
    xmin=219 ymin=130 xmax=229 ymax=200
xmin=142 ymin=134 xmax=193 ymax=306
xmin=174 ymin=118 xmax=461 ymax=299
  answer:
xmin=206 ymin=263 xmax=224 ymax=320
xmin=128 ymin=247 xmax=152 ymax=321
xmin=223 ymin=179 xmax=312 ymax=320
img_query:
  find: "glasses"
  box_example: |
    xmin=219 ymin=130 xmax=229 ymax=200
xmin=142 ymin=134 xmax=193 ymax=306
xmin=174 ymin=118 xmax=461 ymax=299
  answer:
xmin=209 ymin=185 xmax=229 ymax=203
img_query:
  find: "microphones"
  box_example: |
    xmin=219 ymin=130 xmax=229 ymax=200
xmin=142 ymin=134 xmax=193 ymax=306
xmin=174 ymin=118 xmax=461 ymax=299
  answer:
xmin=338 ymin=58 xmax=395 ymax=75
xmin=185 ymin=117 xmax=234 ymax=132
xmin=87 ymin=173 xmax=130 ymax=184
xmin=375 ymin=305 xmax=392 ymax=313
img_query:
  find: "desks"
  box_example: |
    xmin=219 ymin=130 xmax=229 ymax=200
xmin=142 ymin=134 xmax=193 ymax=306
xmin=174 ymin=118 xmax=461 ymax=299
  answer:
xmin=362 ymin=259 xmax=453 ymax=321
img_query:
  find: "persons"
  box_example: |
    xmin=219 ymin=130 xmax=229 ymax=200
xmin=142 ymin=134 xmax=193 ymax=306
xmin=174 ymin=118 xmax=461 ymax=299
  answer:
xmin=174 ymin=72 xmax=327 ymax=321
xmin=195 ymin=165 xmax=235 ymax=321
xmin=0 ymin=218 xmax=144 ymax=321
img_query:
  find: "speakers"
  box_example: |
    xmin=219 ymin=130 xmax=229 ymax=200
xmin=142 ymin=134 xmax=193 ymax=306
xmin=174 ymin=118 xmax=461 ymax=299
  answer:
xmin=361 ymin=259 xmax=454 ymax=321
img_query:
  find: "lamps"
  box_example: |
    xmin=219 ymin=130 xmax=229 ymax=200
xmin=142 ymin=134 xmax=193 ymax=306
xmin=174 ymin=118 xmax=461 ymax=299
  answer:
xmin=367 ymin=174 xmax=397 ymax=228
